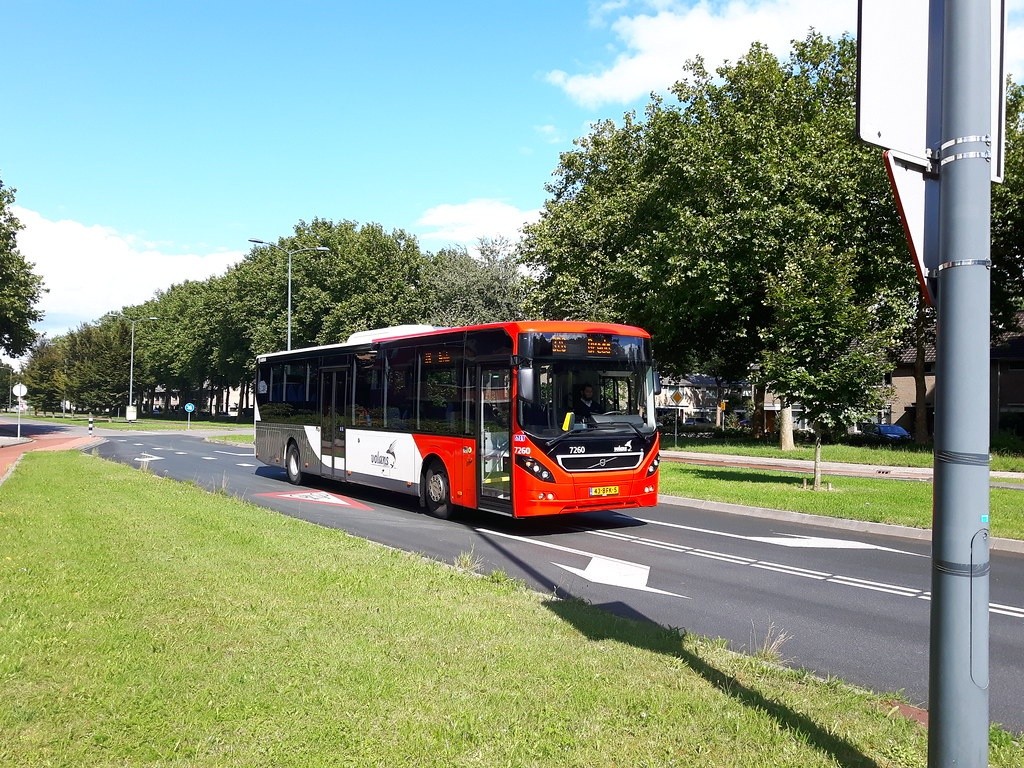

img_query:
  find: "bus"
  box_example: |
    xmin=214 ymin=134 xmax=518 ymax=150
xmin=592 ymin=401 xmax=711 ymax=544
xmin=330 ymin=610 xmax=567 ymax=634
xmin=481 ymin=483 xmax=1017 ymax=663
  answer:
xmin=253 ymin=320 xmax=662 ymax=522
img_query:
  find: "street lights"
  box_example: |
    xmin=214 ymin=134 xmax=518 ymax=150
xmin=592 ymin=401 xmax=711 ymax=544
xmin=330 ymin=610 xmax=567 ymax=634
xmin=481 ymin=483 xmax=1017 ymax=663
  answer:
xmin=248 ymin=238 xmax=330 ymax=374
xmin=109 ymin=314 xmax=161 ymax=423
xmin=0 ymin=367 xmax=13 ymax=413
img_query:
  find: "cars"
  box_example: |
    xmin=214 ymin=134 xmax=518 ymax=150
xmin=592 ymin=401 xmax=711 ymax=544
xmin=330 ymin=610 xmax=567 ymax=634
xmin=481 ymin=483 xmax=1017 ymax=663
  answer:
xmin=864 ymin=422 xmax=912 ymax=447
xmin=738 ymin=420 xmax=753 ymax=429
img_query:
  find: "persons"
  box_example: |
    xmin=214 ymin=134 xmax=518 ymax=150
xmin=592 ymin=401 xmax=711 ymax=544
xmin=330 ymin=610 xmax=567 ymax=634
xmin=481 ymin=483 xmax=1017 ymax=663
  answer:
xmin=574 ymin=385 xmax=604 ymax=423
xmin=359 ymin=409 xmax=371 ymax=427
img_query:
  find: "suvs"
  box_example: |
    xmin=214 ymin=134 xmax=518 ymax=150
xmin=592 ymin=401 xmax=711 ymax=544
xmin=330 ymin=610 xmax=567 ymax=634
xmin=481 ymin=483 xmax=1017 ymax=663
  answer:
xmin=685 ymin=416 xmax=716 ymax=428
